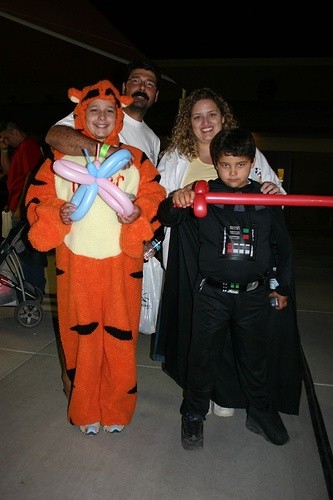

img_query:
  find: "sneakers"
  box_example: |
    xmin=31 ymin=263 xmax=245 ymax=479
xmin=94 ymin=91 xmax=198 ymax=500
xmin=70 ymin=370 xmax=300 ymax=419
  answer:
xmin=181 ymin=413 xmax=203 ymax=450
xmin=246 ymin=412 xmax=291 ymax=445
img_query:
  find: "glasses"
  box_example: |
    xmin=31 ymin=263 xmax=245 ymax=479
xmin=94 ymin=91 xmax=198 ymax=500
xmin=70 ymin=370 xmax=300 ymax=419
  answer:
xmin=128 ymin=77 xmax=157 ymax=88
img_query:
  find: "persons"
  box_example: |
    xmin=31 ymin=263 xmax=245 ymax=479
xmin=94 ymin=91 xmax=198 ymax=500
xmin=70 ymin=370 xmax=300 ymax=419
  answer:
xmin=47 ymin=60 xmax=163 ymax=403
xmin=24 ymin=78 xmax=167 ymax=434
xmin=156 ymin=130 xmax=303 ymax=451
xmin=155 ymin=90 xmax=286 ymax=418
xmin=0 ymin=117 xmax=47 ymax=305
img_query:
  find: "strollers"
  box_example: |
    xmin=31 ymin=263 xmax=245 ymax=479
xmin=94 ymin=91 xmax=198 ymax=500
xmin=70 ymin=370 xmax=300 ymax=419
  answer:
xmin=0 ymin=213 xmax=45 ymax=329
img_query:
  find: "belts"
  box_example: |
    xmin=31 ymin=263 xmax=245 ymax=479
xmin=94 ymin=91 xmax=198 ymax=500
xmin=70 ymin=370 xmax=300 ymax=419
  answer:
xmin=205 ymin=277 xmax=267 ymax=293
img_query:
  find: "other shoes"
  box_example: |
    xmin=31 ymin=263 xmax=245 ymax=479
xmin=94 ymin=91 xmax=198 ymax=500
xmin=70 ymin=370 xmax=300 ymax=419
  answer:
xmin=214 ymin=402 xmax=235 ymax=416
xmin=79 ymin=422 xmax=100 ymax=436
xmin=103 ymin=424 xmax=124 ymax=434
xmin=205 ymin=400 xmax=212 ymax=417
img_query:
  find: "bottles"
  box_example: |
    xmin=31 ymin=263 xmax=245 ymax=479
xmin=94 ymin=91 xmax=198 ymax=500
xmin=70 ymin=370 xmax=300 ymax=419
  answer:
xmin=144 ymin=238 xmax=162 ymax=261
xmin=269 ymin=267 xmax=280 ymax=307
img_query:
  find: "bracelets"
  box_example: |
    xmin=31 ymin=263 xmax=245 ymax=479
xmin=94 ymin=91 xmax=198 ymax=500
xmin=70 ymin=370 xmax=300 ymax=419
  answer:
xmin=1 ymin=149 xmax=8 ymax=153
xmin=99 ymin=144 xmax=111 ymax=159
